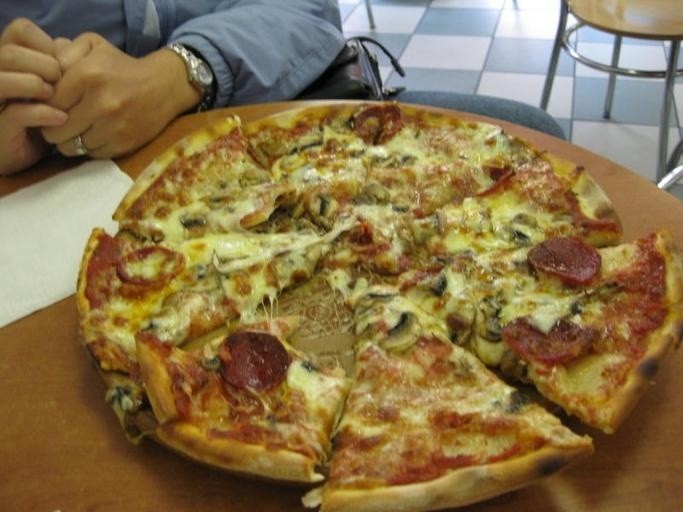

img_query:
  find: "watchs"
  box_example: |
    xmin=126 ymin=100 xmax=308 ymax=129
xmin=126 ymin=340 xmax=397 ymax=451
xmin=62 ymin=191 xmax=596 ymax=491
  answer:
xmin=162 ymin=40 xmax=215 ymax=109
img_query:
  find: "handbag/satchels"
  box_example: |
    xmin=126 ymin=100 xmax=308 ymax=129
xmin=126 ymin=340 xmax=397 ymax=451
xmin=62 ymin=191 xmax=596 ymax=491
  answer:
xmin=293 ymin=37 xmax=405 ymax=99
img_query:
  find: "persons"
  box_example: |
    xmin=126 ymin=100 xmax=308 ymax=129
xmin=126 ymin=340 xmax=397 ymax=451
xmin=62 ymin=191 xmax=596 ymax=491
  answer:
xmin=1 ymin=1 xmax=346 ymax=180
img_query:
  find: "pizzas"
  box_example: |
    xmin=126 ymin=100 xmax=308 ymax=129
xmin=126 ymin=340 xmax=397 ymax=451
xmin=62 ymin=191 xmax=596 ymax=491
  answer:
xmin=73 ymin=99 xmax=683 ymax=512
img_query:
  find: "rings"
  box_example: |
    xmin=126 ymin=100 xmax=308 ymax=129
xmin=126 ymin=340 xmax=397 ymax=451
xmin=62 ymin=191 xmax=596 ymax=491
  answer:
xmin=73 ymin=133 xmax=89 ymax=158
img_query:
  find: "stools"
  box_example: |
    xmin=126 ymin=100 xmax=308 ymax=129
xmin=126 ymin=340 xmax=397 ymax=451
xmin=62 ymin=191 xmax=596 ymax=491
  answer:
xmin=541 ymin=0 xmax=683 ymax=187
